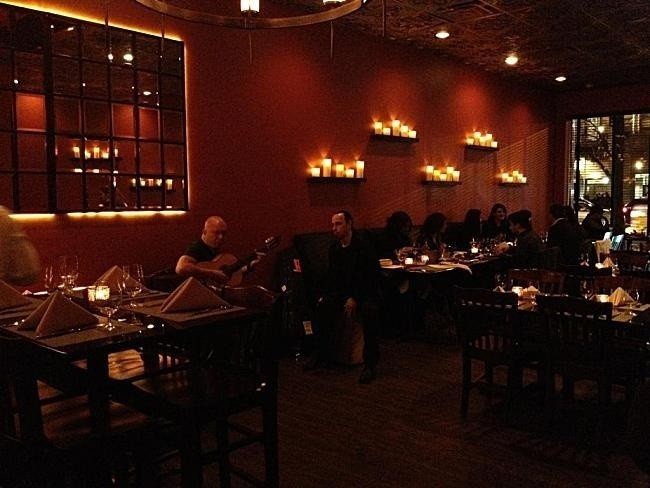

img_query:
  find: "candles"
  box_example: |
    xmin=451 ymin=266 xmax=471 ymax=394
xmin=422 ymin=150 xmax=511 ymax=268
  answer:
xmin=304 ymin=115 xmax=529 ymax=190
xmin=73 ymin=141 xmax=185 ymax=192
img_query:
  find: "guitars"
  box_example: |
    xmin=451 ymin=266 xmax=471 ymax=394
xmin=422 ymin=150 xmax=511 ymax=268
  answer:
xmin=195 ymin=236 xmax=282 ymax=288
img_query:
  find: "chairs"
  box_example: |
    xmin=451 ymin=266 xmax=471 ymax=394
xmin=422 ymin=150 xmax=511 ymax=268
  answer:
xmin=454 ymin=236 xmax=648 ymax=431
xmin=293 ymin=222 xmax=509 ymax=359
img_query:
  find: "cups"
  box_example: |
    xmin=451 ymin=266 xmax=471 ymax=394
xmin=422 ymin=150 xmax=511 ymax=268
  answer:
xmin=471 ymin=241 xmax=478 ymax=254
xmin=512 ymin=286 xmax=523 ymax=297
xmin=596 ymin=294 xmax=609 ymax=302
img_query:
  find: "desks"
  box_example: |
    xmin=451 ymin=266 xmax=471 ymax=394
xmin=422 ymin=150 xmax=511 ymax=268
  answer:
xmin=2 ymin=260 xmax=293 ymax=486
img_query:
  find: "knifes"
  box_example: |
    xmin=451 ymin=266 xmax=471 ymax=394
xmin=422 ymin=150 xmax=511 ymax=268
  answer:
xmin=187 ymin=305 xmax=232 ymax=317
xmin=35 ymin=323 xmax=106 ymax=340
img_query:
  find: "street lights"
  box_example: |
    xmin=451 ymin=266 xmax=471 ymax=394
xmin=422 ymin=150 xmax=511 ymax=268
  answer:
xmin=574 ymin=118 xmax=608 ymax=205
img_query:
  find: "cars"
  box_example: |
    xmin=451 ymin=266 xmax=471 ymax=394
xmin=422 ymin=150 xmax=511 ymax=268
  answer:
xmin=571 ymin=197 xmax=611 ymax=228
xmin=622 ymin=195 xmax=648 ymax=233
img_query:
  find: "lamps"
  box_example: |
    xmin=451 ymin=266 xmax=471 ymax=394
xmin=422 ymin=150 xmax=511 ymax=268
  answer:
xmin=138 ymin=0 xmax=370 ymax=31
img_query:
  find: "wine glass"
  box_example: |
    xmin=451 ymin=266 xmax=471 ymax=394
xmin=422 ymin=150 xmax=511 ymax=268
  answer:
xmin=398 ymin=252 xmax=406 ymax=266
xmin=116 ymin=263 xmax=144 ymax=324
xmin=623 ymin=290 xmax=640 ymax=319
xmin=580 ymin=280 xmax=593 ymax=300
xmin=98 ymin=280 xmax=123 ymax=330
xmin=43 ymin=255 xmax=79 ymax=301
